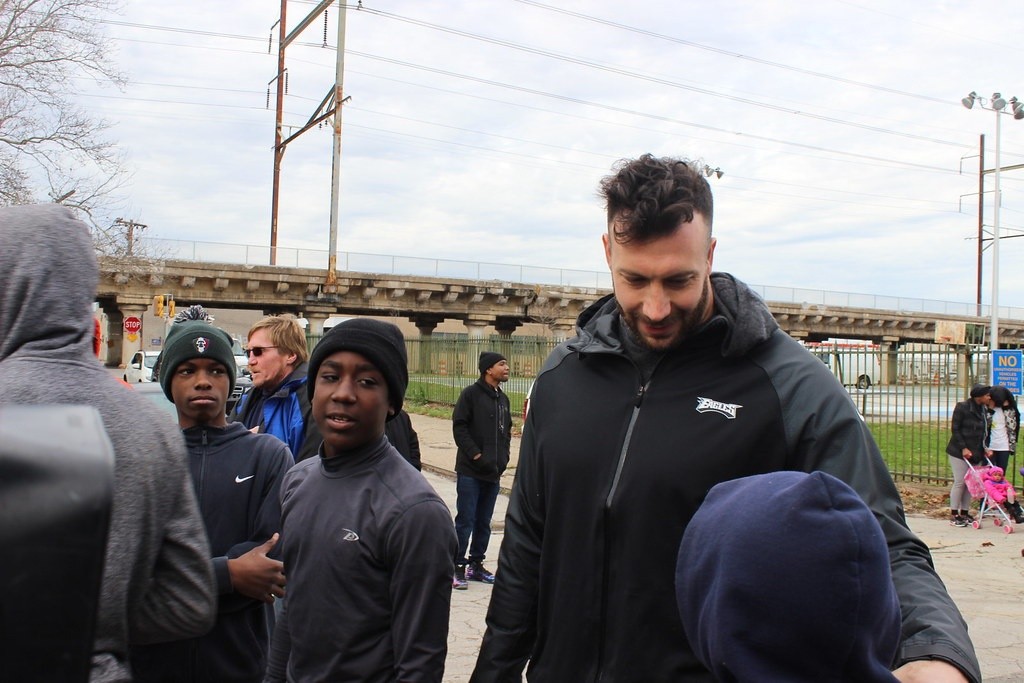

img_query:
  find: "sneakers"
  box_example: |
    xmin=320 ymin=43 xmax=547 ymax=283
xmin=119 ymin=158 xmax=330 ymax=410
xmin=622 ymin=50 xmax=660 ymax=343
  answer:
xmin=452 ymin=564 xmax=467 ymax=588
xmin=466 ymin=562 xmax=496 ymax=584
xmin=949 ymin=514 xmax=968 ymax=527
xmin=959 ymin=515 xmax=974 ymax=524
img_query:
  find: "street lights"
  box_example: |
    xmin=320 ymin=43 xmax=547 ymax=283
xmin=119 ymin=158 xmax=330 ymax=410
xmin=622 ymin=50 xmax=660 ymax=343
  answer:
xmin=963 ymin=90 xmax=1024 ymax=386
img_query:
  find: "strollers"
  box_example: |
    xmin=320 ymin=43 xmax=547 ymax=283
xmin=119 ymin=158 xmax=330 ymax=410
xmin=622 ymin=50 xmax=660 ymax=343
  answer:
xmin=963 ymin=451 xmax=1024 ymax=534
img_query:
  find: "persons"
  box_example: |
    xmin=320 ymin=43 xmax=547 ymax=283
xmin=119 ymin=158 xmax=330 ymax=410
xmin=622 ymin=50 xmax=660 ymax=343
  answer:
xmin=452 ymin=351 xmax=512 ymax=589
xmin=266 ymin=318 xmax=460 ymax=682
xmin=0 ymin=201 xmax=216 ymax=683
xmin=980 ymin=386 xmax=1016 ymax=511
xmin=675 ymin=471 xmax=903 ymax=683
xmin=386 ymin=408 xmax=421 ymax=472
xmin=467 ymin=153 xmax=983 ymax=683
xmin=225 ymin=315 xmax=322 ymax=463
xmin=93 ymin=317 xmax=134 ymax=391
xmin=946 ymin=385 xmax=993 ymax=527
xmin=133 ymin=305 xmax=296 ymax=683
xmin=985 ymin=467 xmax=1024 ymax=524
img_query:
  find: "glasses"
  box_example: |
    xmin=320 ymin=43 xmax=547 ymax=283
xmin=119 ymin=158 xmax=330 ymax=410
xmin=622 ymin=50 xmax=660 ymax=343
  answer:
xmin=246 ymin=346 xmax=279 ymax=357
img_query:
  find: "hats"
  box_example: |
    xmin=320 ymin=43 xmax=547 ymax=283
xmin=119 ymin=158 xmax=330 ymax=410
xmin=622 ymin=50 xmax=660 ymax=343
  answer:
xmin=158 ymin=305 xmax=236 ymax=403
xmin=478 ymin=352 xmax=507 ymax=375
xmin=988 ymin=467 xmax=1003 ymax=476
xmin=307 ymin=318 xmax=408 ymax=423
xmin=970 ymin=384 xmax=990 ymax=397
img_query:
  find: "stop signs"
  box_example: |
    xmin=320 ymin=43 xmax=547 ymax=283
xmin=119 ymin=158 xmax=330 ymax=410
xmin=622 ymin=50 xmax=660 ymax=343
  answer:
xmin=124 ymin=316 xmax=141 ymax=334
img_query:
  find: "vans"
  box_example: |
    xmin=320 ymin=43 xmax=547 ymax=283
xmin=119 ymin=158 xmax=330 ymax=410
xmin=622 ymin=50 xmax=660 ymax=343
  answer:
xmin=807 ymin=346 xmax=881 ymax=389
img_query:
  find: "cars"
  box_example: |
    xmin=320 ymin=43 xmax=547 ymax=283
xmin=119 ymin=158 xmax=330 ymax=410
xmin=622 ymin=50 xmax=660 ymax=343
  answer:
xmin=123 ymin=350 xmax=162 ymax=383
xmin=225 ymin=338 xmax=254 ymax=416
xmin=910 ymin=362 xmax=988 ymax=387
